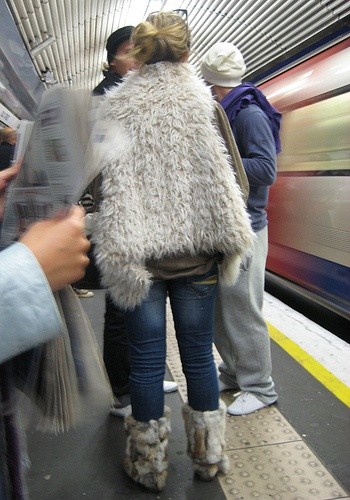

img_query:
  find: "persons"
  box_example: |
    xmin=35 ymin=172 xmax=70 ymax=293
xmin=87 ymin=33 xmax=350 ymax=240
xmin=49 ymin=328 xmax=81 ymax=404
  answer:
xmin=0 ymin=8 xmax=257 ymax=500
xmin=201 ymin=43 xmax=281 ymax=415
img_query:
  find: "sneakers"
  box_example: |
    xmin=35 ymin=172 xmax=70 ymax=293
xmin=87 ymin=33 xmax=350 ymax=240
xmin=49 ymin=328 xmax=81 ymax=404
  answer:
xmin=217 ymin=376 xmax=239 ymax=392
xmin=227 ymin=389 xmax=268 ymax=415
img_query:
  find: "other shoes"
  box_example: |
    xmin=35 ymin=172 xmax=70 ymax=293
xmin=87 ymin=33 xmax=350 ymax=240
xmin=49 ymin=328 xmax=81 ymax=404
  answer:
xmin=74 ymin=288 xmax=94 ymax=298
xmin=163 ymin=380 xmax=178 ymax=392
xmin=111 ymin=403 xmax=133 ymax=416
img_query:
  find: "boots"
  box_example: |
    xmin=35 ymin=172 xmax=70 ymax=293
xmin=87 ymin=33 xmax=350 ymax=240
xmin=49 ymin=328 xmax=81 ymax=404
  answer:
xmin=181 ymin=397 xmax=229 ymax=481
xmin=122 ymin=404 xmax=171 ymax=491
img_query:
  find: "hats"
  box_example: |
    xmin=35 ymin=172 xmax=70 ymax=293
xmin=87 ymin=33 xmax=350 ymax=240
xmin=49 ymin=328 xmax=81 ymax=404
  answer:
xmin=201 ymin=42 xmax=246 ymax=87
xmin=106 ymin=26 xmax=136 ymax=53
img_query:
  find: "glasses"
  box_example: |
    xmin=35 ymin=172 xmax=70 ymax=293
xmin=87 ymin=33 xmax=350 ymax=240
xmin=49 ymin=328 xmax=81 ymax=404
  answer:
xmin=148 ymin=8 xmax=188 ymax=35
